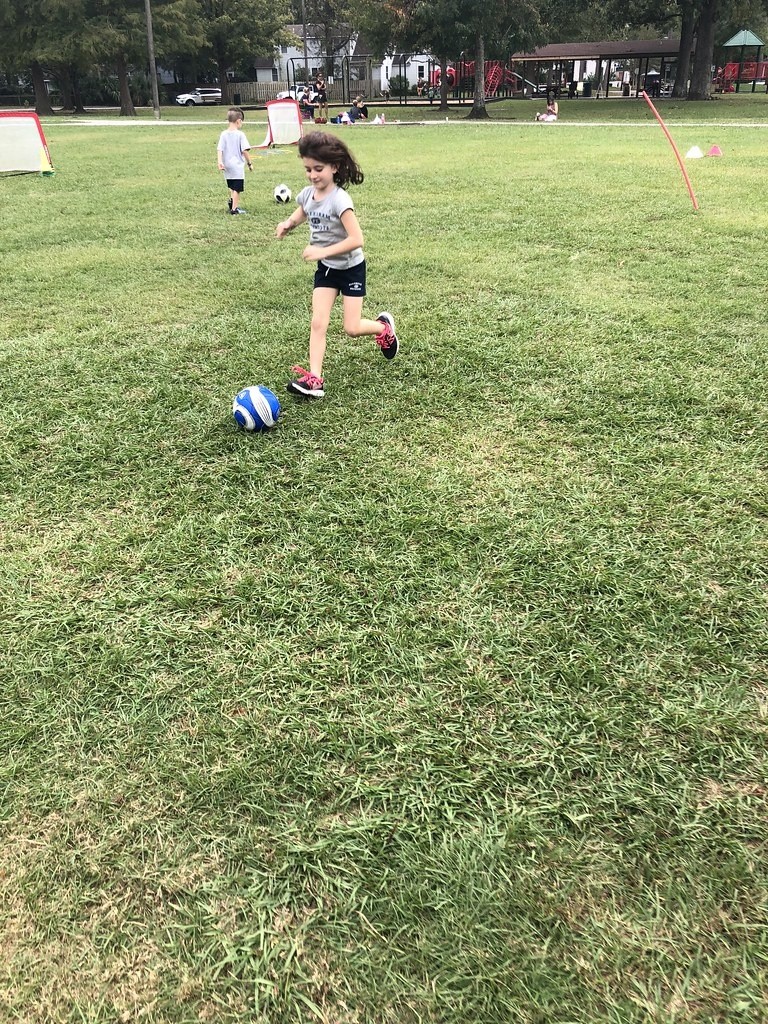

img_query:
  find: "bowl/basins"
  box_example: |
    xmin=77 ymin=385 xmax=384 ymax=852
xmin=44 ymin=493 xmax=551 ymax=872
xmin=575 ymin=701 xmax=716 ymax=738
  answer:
xmin=330 ymin=117 xmax=337 ymax=123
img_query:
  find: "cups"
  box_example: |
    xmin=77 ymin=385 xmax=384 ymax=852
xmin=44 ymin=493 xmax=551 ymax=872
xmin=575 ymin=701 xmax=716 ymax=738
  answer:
xmin=445 ymin=117 xmax=448 ymax=121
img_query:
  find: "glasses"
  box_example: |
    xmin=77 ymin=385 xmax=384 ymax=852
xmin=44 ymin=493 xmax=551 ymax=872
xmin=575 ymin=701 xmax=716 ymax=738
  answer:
xmin=318 ymin=75 xmax=322 ymax=78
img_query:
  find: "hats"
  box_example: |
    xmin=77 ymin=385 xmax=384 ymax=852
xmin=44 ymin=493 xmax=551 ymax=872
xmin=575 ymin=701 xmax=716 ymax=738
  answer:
xmin=303 ymin=88 xmax=309 ymax=93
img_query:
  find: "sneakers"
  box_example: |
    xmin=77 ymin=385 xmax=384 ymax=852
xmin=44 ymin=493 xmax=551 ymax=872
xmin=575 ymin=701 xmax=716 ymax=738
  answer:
xmin=286 ymin=366 xmax=325 ymax=397
xmin=373 ymin=311 xmax=401 ymax=360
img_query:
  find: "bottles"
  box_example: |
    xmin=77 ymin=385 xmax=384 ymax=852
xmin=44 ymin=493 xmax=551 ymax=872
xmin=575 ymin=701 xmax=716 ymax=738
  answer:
xmin=337 ymin=115 xmax=340 ymax=124
xmin=381 ymin=113 xmax=384 ymax=123
xmin=376 ymin=114 xmax=378 ymax=118
xmin=309 ymin=116 xmax=312 ymax=122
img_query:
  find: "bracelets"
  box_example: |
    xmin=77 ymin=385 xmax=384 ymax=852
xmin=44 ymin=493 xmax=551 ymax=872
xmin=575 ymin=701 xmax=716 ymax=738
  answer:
xmin=247 ymin=162 xmax=252 ymax=166
xmin=288 ymin=218 xmax=297 ymax=225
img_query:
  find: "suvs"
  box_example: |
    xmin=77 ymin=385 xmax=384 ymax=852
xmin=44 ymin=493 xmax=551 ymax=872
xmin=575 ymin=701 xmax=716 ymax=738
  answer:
xmin=176 ymin=88 xmax=222 ymax=106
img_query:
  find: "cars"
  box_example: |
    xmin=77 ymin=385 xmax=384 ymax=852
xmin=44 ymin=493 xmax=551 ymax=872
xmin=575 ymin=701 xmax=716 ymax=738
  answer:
xmin=277 ymin=84 xmax=327 ymax=104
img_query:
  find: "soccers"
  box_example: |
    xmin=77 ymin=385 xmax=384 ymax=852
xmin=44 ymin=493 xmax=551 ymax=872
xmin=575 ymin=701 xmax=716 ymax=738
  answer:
xmin=233 ymin=385 xmax=281 ymax=432
xmin=273 ymin=184 xmax=292 ymax=204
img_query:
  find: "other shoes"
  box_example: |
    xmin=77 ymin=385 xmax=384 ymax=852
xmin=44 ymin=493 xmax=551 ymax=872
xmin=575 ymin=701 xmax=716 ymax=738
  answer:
xmin=231 ymin=207 xmax=246 ymax=215
xmin=228 ymin=198 xmax=238 ymax=210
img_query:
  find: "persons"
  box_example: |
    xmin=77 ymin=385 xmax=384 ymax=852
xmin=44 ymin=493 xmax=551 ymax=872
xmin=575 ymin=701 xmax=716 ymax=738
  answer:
xmin=429 ymin=89 xmax=434 ymax=104
xmin=274 ymin=132 xmax=398 ymax=398
xmin=417 ymin=79 xmax=424 ymax=98
xmin=299 ymin=88 xmax=317 ymax=119
xmin=217 ymin=108 xmax=253 ymax=214
xmin=543 ymin=96 xmax=559 ymax=121
xmin=350 ymin=96 xmax=368 ymax=119
xmin=314 ymin=73 xmax=330 ymax=120
